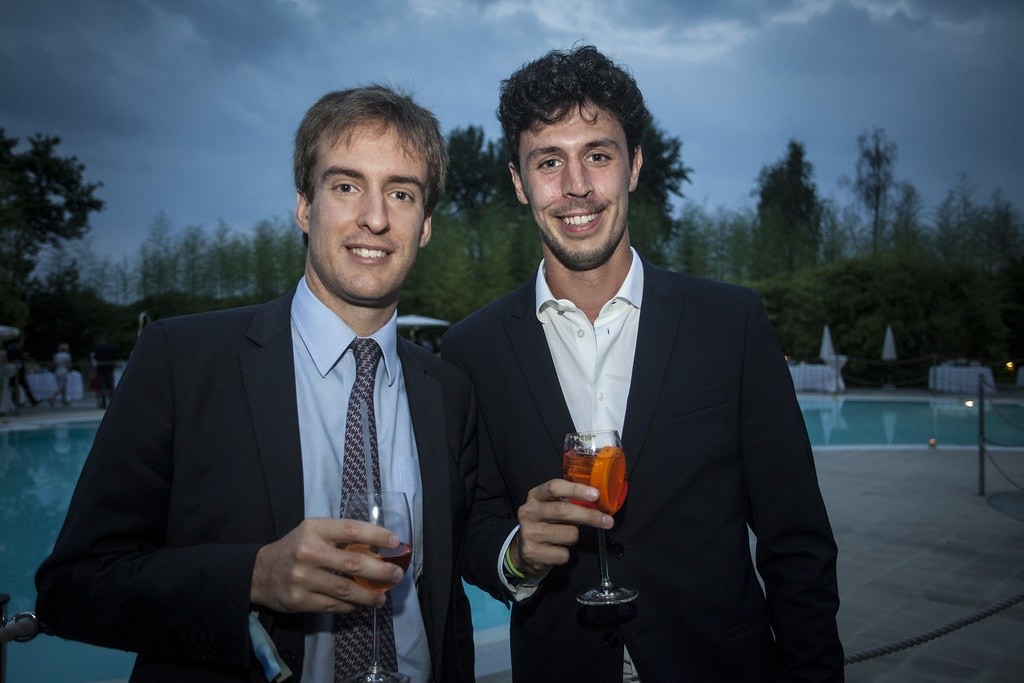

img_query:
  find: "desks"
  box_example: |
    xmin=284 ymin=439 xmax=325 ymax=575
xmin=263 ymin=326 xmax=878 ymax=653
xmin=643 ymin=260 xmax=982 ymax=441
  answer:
xmin=928 ymin=367 xmax=994 ymax=396
xmin=24 ymin=373 xmax=82 ymax=400
xmin=788 ymin=364 xmax=845 ymax=393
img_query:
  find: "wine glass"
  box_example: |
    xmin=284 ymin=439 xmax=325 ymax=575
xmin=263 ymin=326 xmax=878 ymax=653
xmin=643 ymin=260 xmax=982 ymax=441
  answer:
xmin=561 ymin=430 xmax=639 ymax=605
xmin=340 ymin=490 xmax=413 ymax=683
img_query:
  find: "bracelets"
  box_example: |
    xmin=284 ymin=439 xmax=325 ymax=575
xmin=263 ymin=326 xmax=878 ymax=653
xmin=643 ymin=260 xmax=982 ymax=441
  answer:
xmin=504 ymin=547 xmax=525 ymax=577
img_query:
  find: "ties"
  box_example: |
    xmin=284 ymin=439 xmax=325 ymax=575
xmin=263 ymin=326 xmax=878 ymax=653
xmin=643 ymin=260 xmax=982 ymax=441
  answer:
xmin=334 ymin=337 xmax=399 ymax=683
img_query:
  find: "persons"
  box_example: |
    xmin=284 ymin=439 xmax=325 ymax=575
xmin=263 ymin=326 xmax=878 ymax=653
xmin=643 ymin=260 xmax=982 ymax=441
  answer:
xmin=95 ymin=353 xmax=115 ymax=408
xmin=35 ymin=85 xmax=476 ymax=683
xmin=48 ymin=343 xmax=71 ymax=407
xmin=8 ymin=338 xmax=39 ymax=407
xmin=418 ymin=332 xmax=433 ymax=351
xmin=437 ymin=45 xmax=846 ymax=683
